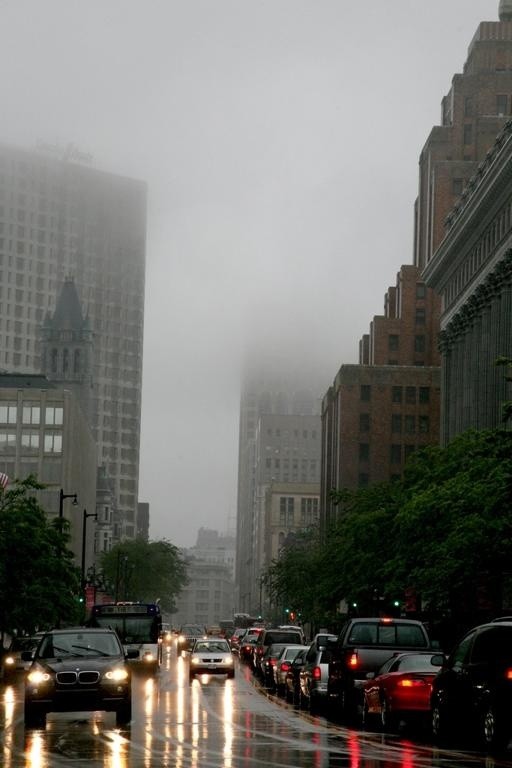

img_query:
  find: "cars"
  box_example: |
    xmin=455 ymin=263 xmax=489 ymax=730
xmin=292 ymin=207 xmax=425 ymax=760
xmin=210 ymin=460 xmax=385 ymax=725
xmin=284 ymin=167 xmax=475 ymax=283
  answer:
xmin=427 ymin=611 xmax=512 ymax=752
xmin=359 ymin=649 xmax=450 ymax=732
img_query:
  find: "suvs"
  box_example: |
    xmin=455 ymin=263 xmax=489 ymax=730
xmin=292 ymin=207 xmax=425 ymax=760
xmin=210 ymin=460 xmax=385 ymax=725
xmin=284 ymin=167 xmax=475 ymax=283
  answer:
xmin=22 ymin=623 xmax=132 ymax=726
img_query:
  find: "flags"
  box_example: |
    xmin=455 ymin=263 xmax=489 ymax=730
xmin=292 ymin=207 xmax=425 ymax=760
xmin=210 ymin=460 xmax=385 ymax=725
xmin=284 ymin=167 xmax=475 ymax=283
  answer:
xmin=0 ymin=472 xmax=9 ymax=490
xmin=102 ymin=595 xmax=113 ymax=605
xmin=86 ymin=587 xmax=95 ymax=609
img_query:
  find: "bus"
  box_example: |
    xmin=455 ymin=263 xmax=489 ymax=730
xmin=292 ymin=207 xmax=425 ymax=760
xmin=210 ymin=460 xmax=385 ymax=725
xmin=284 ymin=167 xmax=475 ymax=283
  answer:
xmin=86 ymin=598 xmax=167 ymax=671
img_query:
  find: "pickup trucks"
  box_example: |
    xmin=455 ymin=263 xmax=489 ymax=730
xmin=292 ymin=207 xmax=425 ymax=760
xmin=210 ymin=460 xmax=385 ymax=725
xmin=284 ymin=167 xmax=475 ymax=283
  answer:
xmin=321 ymin=614 xmax=432 ymax=719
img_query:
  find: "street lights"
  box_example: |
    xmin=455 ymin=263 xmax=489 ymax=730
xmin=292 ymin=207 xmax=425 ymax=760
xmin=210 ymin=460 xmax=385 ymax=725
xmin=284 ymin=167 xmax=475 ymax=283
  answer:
xmin=58 ymin=487 xmax=78 ymax=534
xmin=81 ymin=508 xmax=101 ymax=592
xmin=114 ymin=550 xmax=130 ymax=601
xmin=255 ymin=573 xmax=265 ymax=617
